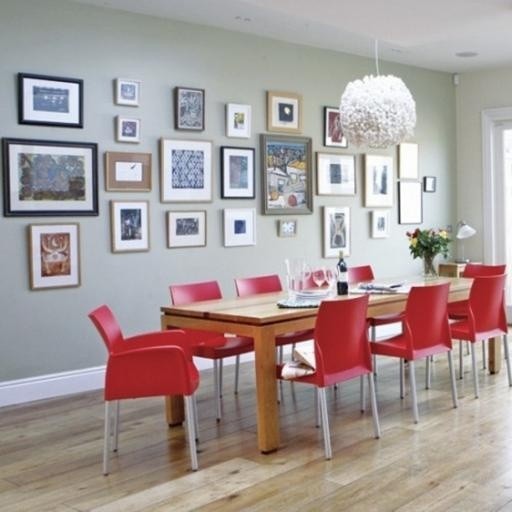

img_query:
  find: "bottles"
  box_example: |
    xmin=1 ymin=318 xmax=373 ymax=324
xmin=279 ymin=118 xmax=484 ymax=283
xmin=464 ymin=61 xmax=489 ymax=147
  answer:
xmin=336 ymin=251 xmax=348 ymax=295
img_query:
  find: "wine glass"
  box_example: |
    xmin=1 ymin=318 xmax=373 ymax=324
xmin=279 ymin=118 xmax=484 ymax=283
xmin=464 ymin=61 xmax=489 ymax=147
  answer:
xmin=312 ymin=264 xmax=324 ymax=295
xmin=325 ymin=266 xmax=338 ymax=298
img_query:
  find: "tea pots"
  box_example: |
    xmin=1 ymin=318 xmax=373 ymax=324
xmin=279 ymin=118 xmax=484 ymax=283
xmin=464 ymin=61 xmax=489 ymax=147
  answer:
xmin=284 ymin=258 xmax=310 ymax=303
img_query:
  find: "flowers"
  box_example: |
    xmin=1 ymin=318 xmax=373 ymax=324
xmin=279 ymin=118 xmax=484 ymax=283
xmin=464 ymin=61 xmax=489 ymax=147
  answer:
xmin=404 ymin=226 xmax=455 ymax=272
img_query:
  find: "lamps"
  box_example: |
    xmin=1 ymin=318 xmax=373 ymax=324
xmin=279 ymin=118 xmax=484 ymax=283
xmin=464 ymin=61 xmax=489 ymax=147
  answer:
xmin=338 ymin=1 xmax=418 ymax=153
xmin=452 ymin=218 xmax=477 ymax=264
